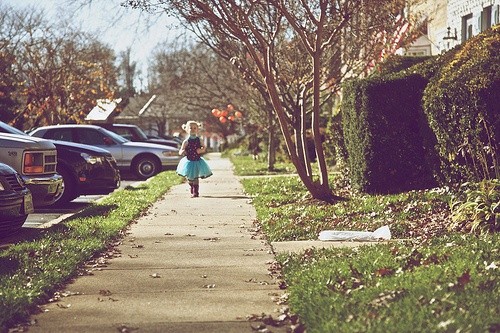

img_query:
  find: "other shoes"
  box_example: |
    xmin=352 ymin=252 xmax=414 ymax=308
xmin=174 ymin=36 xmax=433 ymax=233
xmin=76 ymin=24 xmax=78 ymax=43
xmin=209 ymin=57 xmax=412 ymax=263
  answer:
xmin=190 ymin=194 xmax=199 ymax=198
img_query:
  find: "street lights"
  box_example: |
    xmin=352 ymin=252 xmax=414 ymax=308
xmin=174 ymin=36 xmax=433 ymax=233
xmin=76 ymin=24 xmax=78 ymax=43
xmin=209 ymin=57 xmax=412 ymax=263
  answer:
xmin=442 ymin=25 xmax=458 ymax=49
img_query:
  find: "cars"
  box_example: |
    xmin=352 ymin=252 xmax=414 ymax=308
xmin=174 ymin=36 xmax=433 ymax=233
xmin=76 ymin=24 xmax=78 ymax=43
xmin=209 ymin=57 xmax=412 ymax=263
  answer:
xmin=0 ymin=160 xmax=34 ymax=238
xmin=103 ymin=122 xmax=183 ymax=153
xmin=0 ymin=119 xmax=120 ymax=204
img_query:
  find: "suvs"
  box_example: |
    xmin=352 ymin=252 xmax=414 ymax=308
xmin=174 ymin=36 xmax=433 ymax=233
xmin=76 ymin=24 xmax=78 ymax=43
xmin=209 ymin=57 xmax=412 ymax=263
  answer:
xmin=29 ymin=122 xmax=187 ymax=177
xmin=0 ymin=118 xmax=64 ymax=209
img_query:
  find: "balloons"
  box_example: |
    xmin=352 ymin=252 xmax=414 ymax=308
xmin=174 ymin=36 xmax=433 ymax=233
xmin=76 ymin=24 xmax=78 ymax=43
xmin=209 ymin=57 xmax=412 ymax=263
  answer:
xmin=212 ymin=104 xmax=241 ymax=123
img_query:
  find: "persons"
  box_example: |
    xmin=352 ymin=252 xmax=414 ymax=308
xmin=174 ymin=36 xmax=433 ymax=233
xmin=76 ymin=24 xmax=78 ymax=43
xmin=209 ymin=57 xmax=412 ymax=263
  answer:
xmin=176 ymin=120 xmax=214 ymax=198
